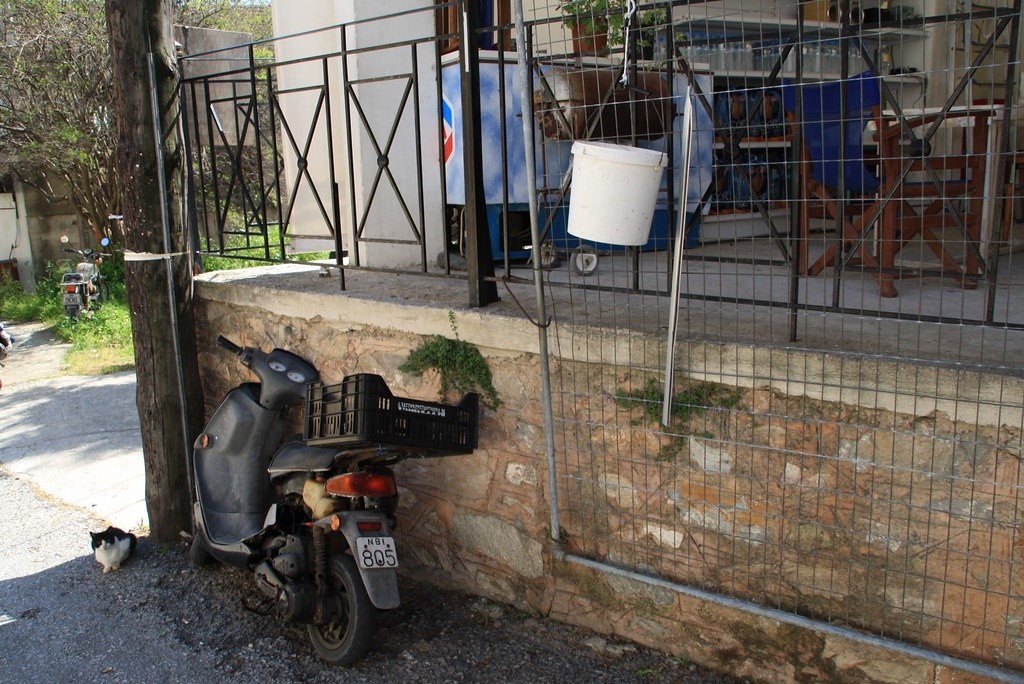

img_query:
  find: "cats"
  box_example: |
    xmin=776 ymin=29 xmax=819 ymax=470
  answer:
xmin=89 ymin=526 xmax=137 ymax=574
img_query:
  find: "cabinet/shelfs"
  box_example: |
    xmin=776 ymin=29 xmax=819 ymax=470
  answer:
xmin=655 ymin=0 xmax=932 ymax=243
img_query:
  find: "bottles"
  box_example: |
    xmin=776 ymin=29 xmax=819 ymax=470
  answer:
xmin=655 ymin=33 xmax=891 ymax=76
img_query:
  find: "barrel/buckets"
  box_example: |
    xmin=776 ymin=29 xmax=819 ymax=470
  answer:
xmin=567 ymin=87 xmax=668 ymax=246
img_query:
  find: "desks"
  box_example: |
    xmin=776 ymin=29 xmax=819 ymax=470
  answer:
xmin=863 ymin=104 xmax=1024 ymax=280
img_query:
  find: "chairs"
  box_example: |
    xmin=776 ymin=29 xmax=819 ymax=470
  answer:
xmin=785 ymin=109 xmax=997 ymax=298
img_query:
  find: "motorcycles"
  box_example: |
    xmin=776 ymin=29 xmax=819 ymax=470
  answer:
xmin=60 ymin=234 xmax=112 ymax=325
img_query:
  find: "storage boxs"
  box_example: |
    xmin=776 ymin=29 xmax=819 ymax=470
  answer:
xmin=303 ymin=374 xmax=478 ymax=459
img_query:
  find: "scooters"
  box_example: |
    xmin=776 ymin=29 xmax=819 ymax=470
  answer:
xmin=190 ymin=336 xmax=412 ymax=667
